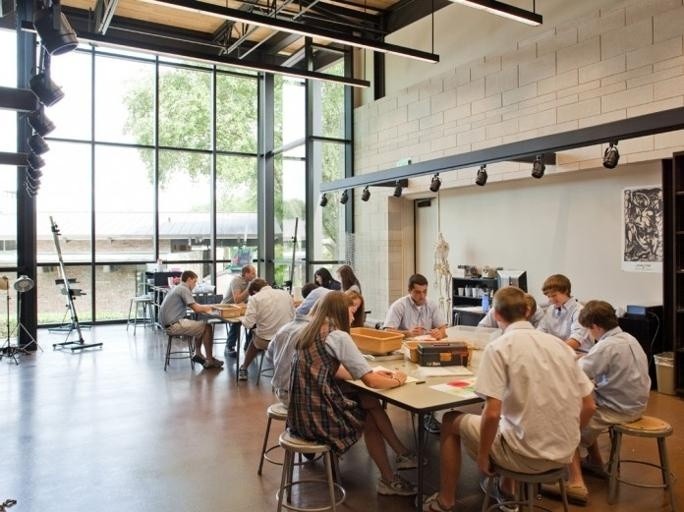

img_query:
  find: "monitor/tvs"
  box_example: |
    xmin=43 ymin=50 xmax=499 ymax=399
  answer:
xmin=496 ymin=270 xmax=527 ymax=294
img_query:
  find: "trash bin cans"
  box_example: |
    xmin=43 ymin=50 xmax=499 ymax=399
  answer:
xmin=653 ymin=352 xmax=678 ymax=395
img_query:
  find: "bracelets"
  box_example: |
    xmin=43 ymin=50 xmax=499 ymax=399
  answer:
xmin=392 ymin=377 xmax=401 ymax=386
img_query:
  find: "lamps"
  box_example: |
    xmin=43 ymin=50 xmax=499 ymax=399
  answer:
xmin=24 ymin=0 xmax=80 ymax=199
xmin=602 ymin=145 xmax=620 ymax=169
xmin=320 ymin=191 xmax=328 ymax=206
xmin=429 ymin=172 xmax=441 ymax=192
xmin=339 ymin=189 xmax=349 ymax=204
xmin=361 ymin=185 xmax=371 ymax=202
xmin=531 ymin=154 xmax=545 ymax=178
xmin=393 ymin=182 xmax=403 ymax=198
xmin=0 ymin=273 xmax=43 ymax=365
xmin=475 ymin=164 xmax=488 ymax=186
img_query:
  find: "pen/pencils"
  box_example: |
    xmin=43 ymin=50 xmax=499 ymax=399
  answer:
xmin=416 ymin=380 xmax=426 ymax=384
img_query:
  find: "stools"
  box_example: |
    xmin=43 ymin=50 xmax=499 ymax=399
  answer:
xmin=482 ymin=454 xmax=569 ymax=512
xmin=208 ymin=318 xmax=229 ymax=344
xmin=126 ymin=296 xmax=155 ymax=335
xmin=275 ymin=430 xmax=347 ymax=511
xmin=257 ymin=402 xmax=324 ymax=475
xmin=163 ymin=333 xmax=196 ymax=371
xmin=256 ymin=348 xmax=273 ymax=385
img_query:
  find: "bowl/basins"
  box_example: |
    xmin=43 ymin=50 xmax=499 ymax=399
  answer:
xmin=147 ymin=279 xmax=154 ymax=284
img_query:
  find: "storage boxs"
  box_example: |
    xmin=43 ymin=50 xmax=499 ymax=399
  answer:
xmin=209 ymin=302 xmax=247 ymax=318
xmin=350 ymin=326 xmax=406 ymax=353
xmin=415 ymin=340 xmax=470 ymax=368
xmin=445 ymin=324 xmax=502 ymax=349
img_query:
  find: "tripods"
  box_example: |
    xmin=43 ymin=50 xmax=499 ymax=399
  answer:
xmin=0 ymin=293 xmax=44 ymax=355
xmin=0 ymin=279 xmax=32 ymax=365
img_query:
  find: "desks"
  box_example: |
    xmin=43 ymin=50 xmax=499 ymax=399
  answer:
xmin=224 ymin=310 xmax=371 ymax=387
xmin=607 ymin=414 xmax=678 ymax=512
xmin=344 ymin=334 xmax=486 ymax=512
xmin=617 ymin=305 xmax=663 ymax=391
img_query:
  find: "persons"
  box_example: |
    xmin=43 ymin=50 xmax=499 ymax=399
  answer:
xmin=417 ymin=285 xmax=597 ymax=512
xmin=284 ymin=290 xmax=432 ymax=496
xmin=381 ymin=274 xmax=448 ymax=434
xmin=157 ymin=269 xmax=224 ymax=369
xmin=221 ymin=263 xmax=367 ymax=461
xmin=540 ymin=300 xmax=652 ymax=503
xmin=537 ymin=273 xmax=597 ymax=352
xmin=477 ymin=294 xmax=545 ymax=330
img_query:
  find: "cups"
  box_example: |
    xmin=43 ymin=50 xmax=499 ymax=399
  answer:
xmin=482 ymin=296 xmax=488 ymax=311
xmin=167 ymin=277 xmax=173 ymax=287
xmin=457 ymin=287 xmax=484 ymax=299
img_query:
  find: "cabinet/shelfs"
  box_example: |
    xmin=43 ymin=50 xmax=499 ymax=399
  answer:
xmin=451 ymin=275 xmax=497 ymax=326
xmin=144 ymin=271 xmax=182 ymax=329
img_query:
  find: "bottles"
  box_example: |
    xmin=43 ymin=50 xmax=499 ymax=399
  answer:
xmin=489 ymin=288 xmax=493 ymax=298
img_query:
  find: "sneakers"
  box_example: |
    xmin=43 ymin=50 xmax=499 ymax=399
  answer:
xmin=192 ymin=355 xmax=224 ymax=368
xmin=414 ymin=492 xmax=456 ymax=512
xmin=239 ymin=368 xmax=248 ymax=379
xmin=375 ymin=446 xmax=431 ymax=497
xmin=540 ymin=479 xmax=590 ymax=506
xmin=225 ymin=345 xmax=236 ymax=357
xmin=480 ymin=474 xmax=520 ymax=512
